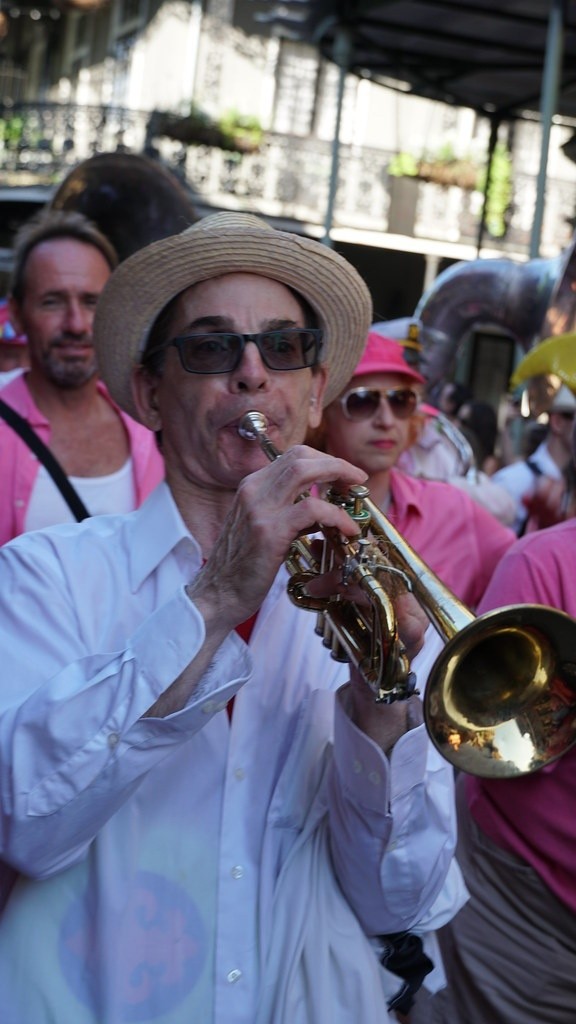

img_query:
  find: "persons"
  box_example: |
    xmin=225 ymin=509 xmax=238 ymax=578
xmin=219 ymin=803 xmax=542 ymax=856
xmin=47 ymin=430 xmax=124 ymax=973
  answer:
xmin=0 ymin=204 xmax=575 ymax=1023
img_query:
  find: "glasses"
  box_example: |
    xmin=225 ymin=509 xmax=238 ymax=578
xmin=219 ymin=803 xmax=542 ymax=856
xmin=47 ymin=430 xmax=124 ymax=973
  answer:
xmin=562 ymin=412 xmax=573 ymax=420
xmin=338 ymin=387 xmax=418 ymax=420
xmin=152 ymin=327 xmax=325 ymax=375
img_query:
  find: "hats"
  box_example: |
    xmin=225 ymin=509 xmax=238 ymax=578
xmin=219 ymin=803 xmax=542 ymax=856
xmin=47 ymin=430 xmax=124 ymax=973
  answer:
xmin=94 ymin=212 xmax=372 ymax=423
xmin=546 ymin=387 xmax=574 ymax=413
xmin=352 ymin=332 xmax=424 ymax=383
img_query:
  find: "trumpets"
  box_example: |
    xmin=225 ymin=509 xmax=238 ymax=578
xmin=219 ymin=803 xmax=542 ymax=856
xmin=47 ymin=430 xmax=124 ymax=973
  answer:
xmin=241 ymin=410 xmax=576 ymax=779
